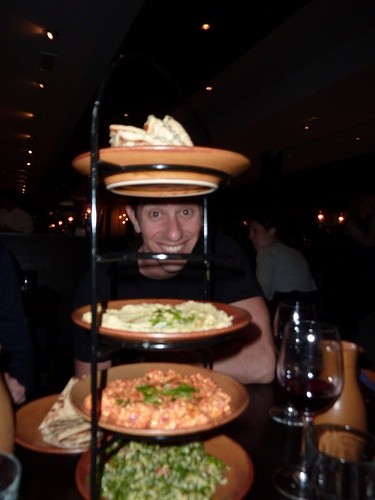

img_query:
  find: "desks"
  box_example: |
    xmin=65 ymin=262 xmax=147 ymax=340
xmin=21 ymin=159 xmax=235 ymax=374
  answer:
xmin=14 ymin=372 xmax=375 ymax=500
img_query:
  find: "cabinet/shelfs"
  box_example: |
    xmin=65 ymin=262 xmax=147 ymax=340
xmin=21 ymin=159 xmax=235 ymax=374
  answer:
xmin=72 ymin=101 xmax=248 ymax=500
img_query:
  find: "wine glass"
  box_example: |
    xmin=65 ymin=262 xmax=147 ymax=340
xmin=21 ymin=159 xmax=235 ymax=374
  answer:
xmin=276 ymin=321 xmax=350 ymax=500
xmin=266 ymin=302 xmax=322 ymax=426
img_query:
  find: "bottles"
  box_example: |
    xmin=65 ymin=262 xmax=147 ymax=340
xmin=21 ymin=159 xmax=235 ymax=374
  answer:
xmin=301 ymin=339 xmax=367 ymax=464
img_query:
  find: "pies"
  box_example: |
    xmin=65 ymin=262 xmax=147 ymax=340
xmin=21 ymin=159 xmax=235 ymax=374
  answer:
xmin=37 ymin=378 xmax=105 ymax=449
xmin=108 ymin=113 xmax=194 ymax=148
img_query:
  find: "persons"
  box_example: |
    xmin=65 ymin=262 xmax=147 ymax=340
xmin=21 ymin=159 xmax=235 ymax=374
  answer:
xmin=345 ymin=197 xmax=375 ymax=364
xmin=0 ymin=246 xmax=35 ymax=403
xmin=71 ymin=198 xmax=276 ymax=385
xmin=248 ymin=207 xmax=318 ymax=302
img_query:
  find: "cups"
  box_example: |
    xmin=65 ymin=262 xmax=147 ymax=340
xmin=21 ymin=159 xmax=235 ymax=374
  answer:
xmin=0 ymin=452 xmax=21 ymax=500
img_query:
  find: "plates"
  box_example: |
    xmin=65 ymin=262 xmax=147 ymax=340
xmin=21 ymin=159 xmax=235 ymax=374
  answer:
xmin=15 ymin=394 xmax=115 ymax=455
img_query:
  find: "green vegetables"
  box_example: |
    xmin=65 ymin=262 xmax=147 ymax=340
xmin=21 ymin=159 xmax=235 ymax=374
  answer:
xmin=83 ymin=438 xmax=227 ymax=500
xmin=148 ymin=306 xmax=197 ymax=330
xmin=115 ymin=383 xmax=196 ymax=404
